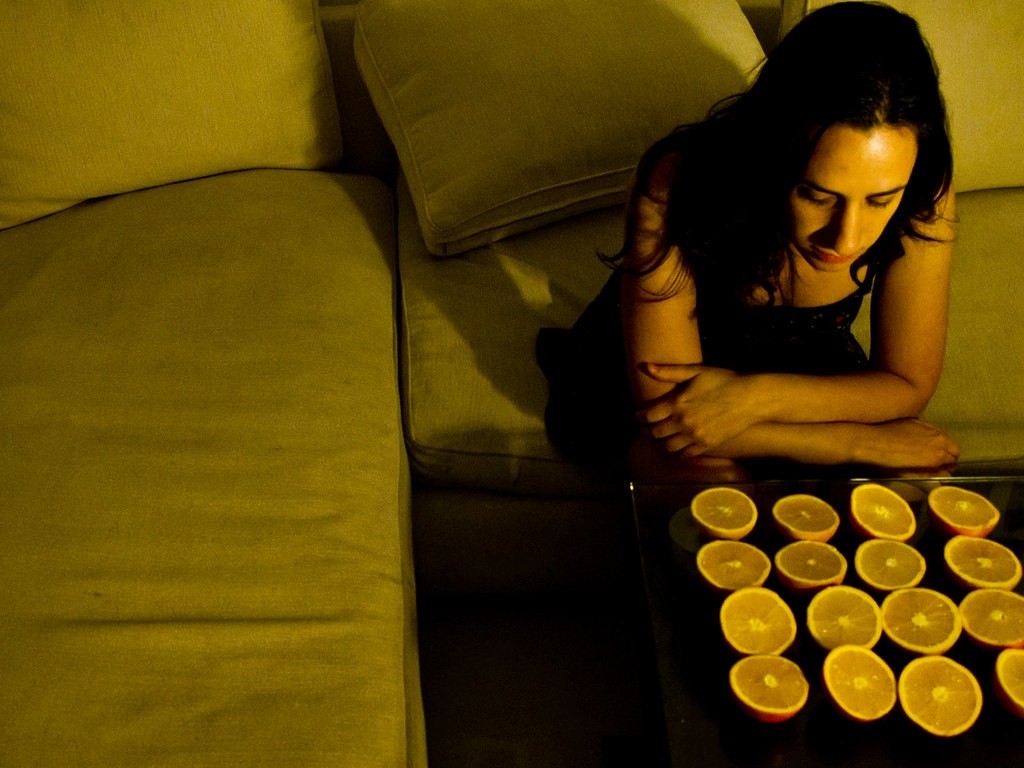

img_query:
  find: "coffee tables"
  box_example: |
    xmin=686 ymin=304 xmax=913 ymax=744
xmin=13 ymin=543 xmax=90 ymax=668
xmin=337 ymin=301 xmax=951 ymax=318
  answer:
xmin=630 ymin=475 xmax=1024 ymax=768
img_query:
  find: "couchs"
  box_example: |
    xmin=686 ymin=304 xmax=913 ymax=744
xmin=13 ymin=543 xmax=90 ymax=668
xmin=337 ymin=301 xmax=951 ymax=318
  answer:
xmin=0 ymin=0 xmax=1024 ymax=768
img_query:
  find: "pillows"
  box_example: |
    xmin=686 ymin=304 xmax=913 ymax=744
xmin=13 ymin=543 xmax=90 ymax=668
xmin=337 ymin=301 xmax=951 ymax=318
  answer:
xmin=776 ymin=0 xmax=1024 ymax=194
xmin=351 ymin=0 xmax=768 ymax=256
xmin=1 ymin=0 xmax=343 ymax=231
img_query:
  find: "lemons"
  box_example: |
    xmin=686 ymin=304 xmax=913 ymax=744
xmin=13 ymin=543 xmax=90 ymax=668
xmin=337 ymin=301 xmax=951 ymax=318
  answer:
xmin=690 ymin=484 xmax=1024 ymax=737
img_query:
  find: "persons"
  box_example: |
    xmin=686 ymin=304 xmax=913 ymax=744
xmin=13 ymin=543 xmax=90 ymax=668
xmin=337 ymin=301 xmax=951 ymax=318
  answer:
xmin=531 ymin=2 xmax=965 ymax=768
